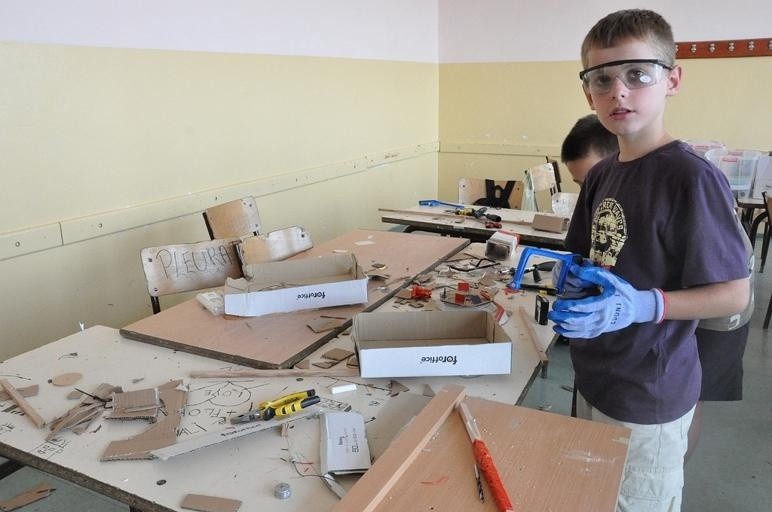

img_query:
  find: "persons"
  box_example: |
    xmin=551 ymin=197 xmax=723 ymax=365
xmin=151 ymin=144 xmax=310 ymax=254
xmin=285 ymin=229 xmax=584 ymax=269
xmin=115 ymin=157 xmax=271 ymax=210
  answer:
xmin=549 ymin=8 xmax=753 ymax=512
xmin=563 ymin=114 xmax=747 ymax=463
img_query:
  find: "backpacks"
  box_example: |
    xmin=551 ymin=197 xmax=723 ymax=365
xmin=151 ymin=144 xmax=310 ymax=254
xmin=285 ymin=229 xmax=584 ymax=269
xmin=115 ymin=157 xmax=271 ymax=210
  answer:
xmin=472 ymin=179 xmax=516 ymax=209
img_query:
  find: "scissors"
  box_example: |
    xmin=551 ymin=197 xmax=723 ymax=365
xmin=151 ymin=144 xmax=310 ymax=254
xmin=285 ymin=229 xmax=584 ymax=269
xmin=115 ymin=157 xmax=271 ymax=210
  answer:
xmin=477 ymin=220 xmax=502 ymax=228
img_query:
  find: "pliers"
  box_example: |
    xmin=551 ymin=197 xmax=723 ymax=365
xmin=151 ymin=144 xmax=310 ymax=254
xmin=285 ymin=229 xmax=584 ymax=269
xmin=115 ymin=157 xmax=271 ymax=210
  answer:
xmin=230 ymin=389 xmax=321 ymax=423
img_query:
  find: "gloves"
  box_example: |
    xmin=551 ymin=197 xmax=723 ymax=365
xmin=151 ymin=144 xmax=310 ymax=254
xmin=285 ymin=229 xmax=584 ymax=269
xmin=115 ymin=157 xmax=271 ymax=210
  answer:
xmin=546 ymin=256 xmax=668 ymax=341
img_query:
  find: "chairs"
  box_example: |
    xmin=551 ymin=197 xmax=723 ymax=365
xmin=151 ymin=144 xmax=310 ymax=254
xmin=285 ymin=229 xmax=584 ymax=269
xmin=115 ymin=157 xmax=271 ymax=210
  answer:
xmin=458 ymin=176 xmax=524 ymax=210
xmin=685 ymin=139 xmax=772 ymax=330
xmin=524 ymin=157 xmax=580 ymax=220
xmin=140 ymin=195 xmax=314 ymax=314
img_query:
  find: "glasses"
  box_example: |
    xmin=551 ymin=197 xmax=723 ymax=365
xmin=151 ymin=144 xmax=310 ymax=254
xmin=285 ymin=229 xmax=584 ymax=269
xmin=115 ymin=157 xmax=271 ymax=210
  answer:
xmin=578 ymin=59 xmax=672 ymax=96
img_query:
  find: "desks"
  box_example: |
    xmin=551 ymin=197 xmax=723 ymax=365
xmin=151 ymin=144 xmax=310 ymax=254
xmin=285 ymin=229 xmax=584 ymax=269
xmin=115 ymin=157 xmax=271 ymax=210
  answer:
xmin=1 ymin=322 xmax=432 ymax=512
xmin=291 ymin=242 xmax=574 ymax=405
xmin=381 ymin=200 xmax=572 ymax=251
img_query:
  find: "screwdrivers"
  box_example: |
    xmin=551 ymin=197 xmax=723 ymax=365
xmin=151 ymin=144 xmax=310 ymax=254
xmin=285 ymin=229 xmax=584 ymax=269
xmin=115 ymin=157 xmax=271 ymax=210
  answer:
xmin=482 ymin=213 xmax=501 ymax=222
